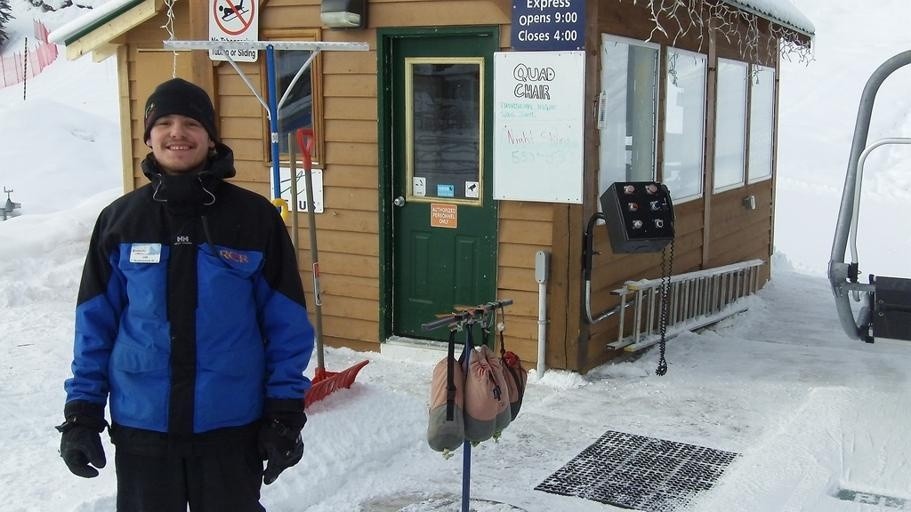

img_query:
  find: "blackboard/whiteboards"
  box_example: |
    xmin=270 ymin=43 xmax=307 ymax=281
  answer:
xmin=493 ymin=50 xmax=586 ymax=205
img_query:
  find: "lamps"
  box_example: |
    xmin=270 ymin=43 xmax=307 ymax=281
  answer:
xmin=318 ymin=0 xmax=367 ymax=31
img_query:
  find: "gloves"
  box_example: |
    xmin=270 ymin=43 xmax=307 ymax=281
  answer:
xmin=255 ymin=398 xmax=309 ymax=486
xmin=54 ymin=400 xmax=107 ymax=478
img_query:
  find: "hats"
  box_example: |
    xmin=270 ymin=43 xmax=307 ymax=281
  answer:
xmin=143 ymin=78 xmax=219 ymax=147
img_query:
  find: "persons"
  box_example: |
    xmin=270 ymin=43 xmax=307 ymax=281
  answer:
xmin=54 ymin=77 xmax=316 ymax=512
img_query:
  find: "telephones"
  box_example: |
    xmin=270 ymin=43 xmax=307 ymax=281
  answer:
xmin=600 ymin=181 xmax=676 ymax=254
